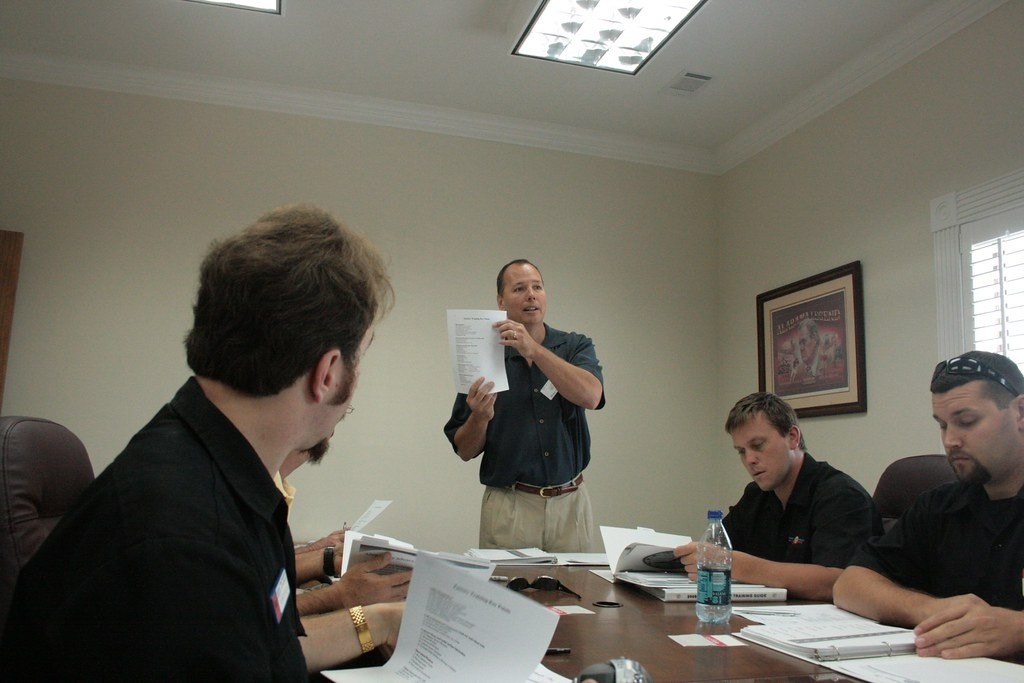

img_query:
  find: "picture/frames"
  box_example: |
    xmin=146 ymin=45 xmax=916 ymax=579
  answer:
xmin=755 ymin=260 xmax=867 ymax=419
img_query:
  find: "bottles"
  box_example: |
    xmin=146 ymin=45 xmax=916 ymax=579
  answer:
xmin=696 ymin=510 xmax=733 ymax=623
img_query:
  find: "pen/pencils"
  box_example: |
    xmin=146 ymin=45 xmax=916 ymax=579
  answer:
xmin=545 ymin=648 xmax=571 ymax=654
xmin=733 ymin=609 xmax=801 ymax=616
xmin=343 ymin=522 xmax=346 ymax=527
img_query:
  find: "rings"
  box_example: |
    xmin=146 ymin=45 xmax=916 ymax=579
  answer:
xmin=514 ymin=331 xmax=516 ymax=339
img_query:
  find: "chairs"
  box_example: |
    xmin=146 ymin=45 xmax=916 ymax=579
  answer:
xmin=0 ymin=416 xmax=94 ymax=626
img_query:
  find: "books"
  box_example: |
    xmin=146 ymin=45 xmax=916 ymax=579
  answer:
xmin=588 ymin=526 xmax=790 ymax=603
xmin=467 ymin=544 xmax=612 ymax=565
xmin=731 ymin=619 xmax=1024 ymax=683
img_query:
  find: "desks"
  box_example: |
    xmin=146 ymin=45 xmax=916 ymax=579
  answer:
xmin=309 ymin=552 xmax=1023 ymax=682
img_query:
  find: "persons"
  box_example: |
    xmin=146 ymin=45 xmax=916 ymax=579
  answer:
xmin=0 ymin=206 xmax=414 ymax=683
xmin=674 ymin=392 xmax=872 ymax=602
xmin=832 ymin=349 xmax=1024 ymax=660
xmin=790 ymin=317 xmax=827 ymax=386
xmin=442 ymin=260 xmax=606 ymax=558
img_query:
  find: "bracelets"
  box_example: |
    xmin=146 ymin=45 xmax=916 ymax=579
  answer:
xmin=349 ymin=605 xmax=374 ymax=653
xmin=323 ymin=546 xmax=335 ymax=576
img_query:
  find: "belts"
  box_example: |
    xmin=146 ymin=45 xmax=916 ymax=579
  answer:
xmin=510 ymin=473 xmax=584 ymax=497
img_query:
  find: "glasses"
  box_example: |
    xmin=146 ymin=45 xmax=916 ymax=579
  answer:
xmin=506 ymin=576 xmax=582 ymax=603
xmin=347 ymin=405 xmax=355 ymax=414
xmin=930 ymin=356 xmax=1019 ymax=398
